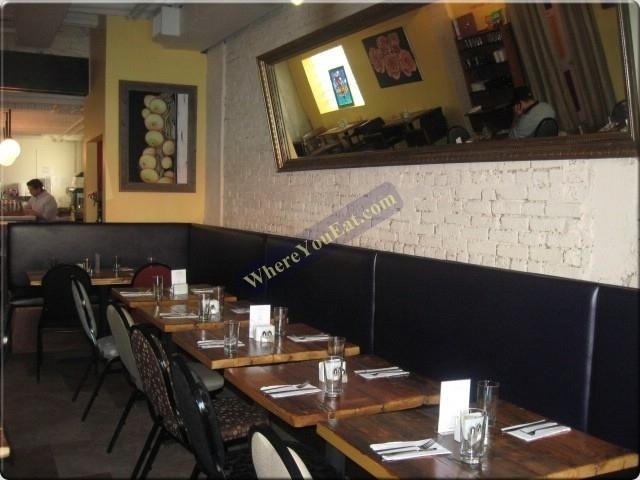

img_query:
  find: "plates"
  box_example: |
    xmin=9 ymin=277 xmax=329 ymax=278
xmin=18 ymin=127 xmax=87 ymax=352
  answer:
xmin=369 ymin=438 xmax=452 ymax=462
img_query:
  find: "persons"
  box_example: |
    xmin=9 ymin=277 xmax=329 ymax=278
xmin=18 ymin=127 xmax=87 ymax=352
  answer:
xmin=21 ymin=179 xmax=58 ymax=222
xmin=506 ymin=84 xmax=557 ymax=137
xmin=334 ymin=71 xmax=346 ymax=99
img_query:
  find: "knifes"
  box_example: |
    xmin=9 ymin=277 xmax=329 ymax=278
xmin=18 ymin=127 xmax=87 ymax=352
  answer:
xmin=502 ymin=417 xmax=551 ymax=433
xmin=197 ymin=339 xmax=245 ymax=348
xmin=264 ymin=388 xmax=323 ymax=396
xmin=156 ymin=310 xmax=197 ymax=320
xmin=352 ymin=366 xmax=411 ymax=379
xmin=291 ymin=332 xmax=333 ymax=341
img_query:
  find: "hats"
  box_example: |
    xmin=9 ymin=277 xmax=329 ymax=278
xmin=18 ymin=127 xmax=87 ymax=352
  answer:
xmin=507 ymin=86 xmax=532 ymax=102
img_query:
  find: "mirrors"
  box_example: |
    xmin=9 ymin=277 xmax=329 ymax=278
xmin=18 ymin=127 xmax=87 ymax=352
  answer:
xmin=254 ymin=2 xmax=640 ymax=172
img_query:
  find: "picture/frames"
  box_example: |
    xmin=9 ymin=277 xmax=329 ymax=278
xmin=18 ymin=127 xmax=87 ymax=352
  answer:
xmin=118 ymin=79 xmax=198 ymax=194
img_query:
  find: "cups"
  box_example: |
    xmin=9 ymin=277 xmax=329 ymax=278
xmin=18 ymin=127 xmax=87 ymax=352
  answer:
xmin=80 ymin=256 xmax=89 ymax=273
xmin=151 ymin=272 xmax=164 ymax=301
xmin=273 ymin=306 xmax=289 ymax=337
xmin=223 ymin=320 xmax=240 ymax=354
xmin=323 ymin=355 xmax=343 ymax=395
xmin=198 ymin=292 xmax=210 ymax=322
xmin=112 ymin=255 xmax=121 ymax=274
xmin=327 ymin=336 xmax=347 ymax=361
xmin=475 ymin=379 xmax=501 ymax=431
xmin=212 ymin=287 xmax=225 ymax=318
xmin=143 ymin=256 xmax=159 ymax=266
xmin=460 ymin=406 xmax=486 ymax=466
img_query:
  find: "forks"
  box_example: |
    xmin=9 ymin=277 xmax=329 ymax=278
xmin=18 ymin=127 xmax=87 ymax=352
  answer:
xmin=259 ymin=382 xmax=310 ymax=393
xmin=192 ymin=286 xmax=219 ymax=292
xmin=520 ymin=424 xmax=574 ymax=438
xmin=116 ymin=288 xmax=152 ymax=298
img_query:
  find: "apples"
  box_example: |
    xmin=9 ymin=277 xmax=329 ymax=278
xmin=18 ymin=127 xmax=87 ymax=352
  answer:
xmin=139 ymin=94 xmax=176 ymax=185
